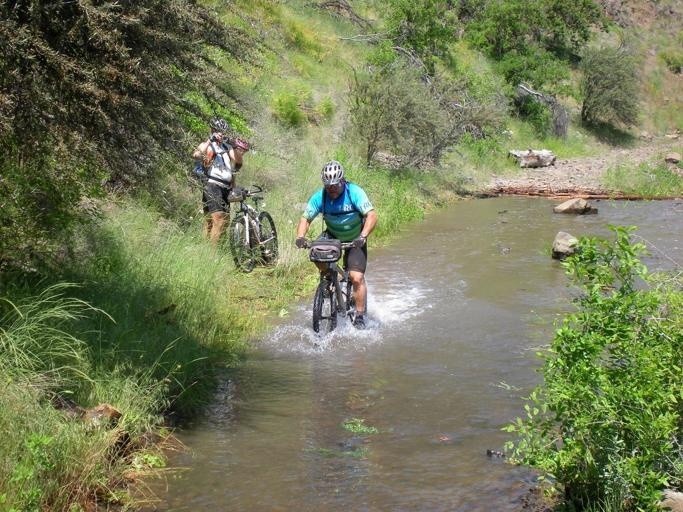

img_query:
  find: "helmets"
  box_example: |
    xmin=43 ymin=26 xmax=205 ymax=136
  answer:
xmin=232 ymin=137 xmax=249 ymax=150
xmin=209 ymin=117 xmax=228 ymax=131
xmin=321 ymin=161 xmax=343 ymax=186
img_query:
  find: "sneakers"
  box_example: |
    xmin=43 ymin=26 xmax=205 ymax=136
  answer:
xmin=353 ymin=315 xmax=366 ymax=330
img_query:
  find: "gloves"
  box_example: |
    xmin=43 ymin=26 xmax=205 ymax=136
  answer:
xmin=296 ymin=237 xmax=306 ymax=249
xmin=353 ymin=235 xmax=367 ymax=248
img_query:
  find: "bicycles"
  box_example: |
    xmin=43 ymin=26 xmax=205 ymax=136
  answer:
xmin=228 ymin=185 xmax=279 ymax=271
xmin=295 ymin=237 xmax=367 ymax=337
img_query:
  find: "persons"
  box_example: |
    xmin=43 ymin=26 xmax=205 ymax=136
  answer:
xmin=193 ymin=117 xmax=249 ymax=244
xmin=295 ymin=161 xmax=377 ymax=331
